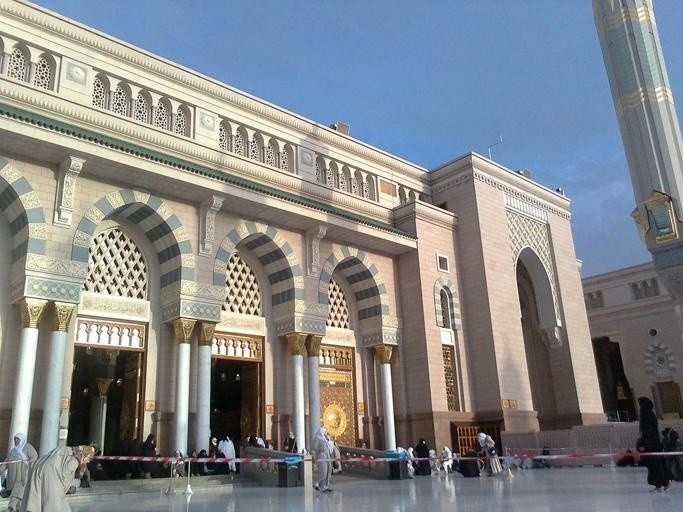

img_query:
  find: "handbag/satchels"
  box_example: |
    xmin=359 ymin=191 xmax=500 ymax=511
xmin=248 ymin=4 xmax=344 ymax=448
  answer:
xmin=488 ymin=447 xmax=498 ymax=457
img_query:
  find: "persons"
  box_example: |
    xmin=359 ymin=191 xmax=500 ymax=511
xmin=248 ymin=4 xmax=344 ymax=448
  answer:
xmin=396 ymin=438 xmax=452 ymax=479
xmin=540 ymin=445 xmax=551 ymax=470
xmin=19 ymin=443 xmax=96 ymax=511
xmin=637 ymin=395 xmax=669 ymax=493
xmin=0 ymin=432 xmax=38 ymax=511
xmin=616 ymin=448 xmax=636 ymax=466
xmin=282 ymin=431 xmax=297 ymax=453
xmin=476 ymin=432 xmax=502 ymax=475
xmin=241 ymin=434 xmax=264 ymax=448
xmin=324 ymin=438 xmax=342 ymax=475
xmin=659 ymin=424 xmax=682 ymax=481
xmin=129 ymin=434 xmax=237 ymax=479
xmin=308 ymin=426 xmax=334 ymax=493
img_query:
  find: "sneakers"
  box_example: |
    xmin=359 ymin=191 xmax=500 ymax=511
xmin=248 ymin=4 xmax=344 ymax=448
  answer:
xmin=312 ymin=484 xmax=333 ymax=494
